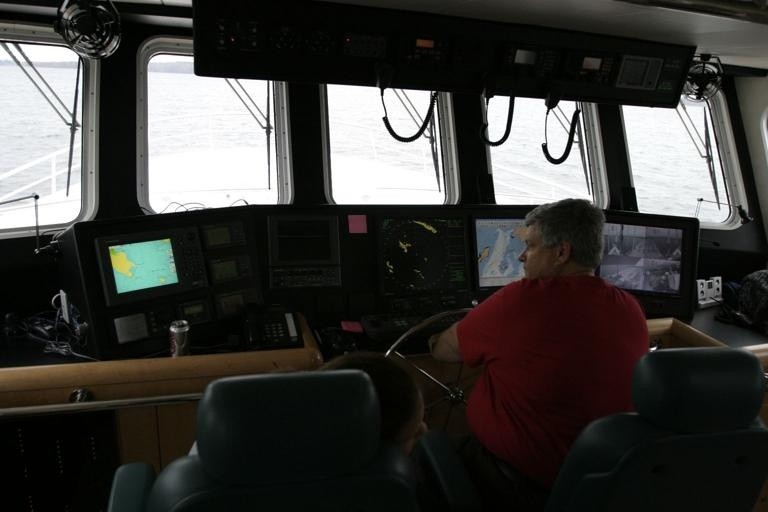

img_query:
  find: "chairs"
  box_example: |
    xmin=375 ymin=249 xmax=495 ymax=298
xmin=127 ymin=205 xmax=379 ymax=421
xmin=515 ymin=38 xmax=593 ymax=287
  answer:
xmin=107 ymin=367 xmax=419 ymax=512
xmin=422 ymin=348 xmax=768 ymax=512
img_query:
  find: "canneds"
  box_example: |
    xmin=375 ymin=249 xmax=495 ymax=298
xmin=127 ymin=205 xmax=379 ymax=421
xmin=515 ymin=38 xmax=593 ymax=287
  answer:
xmin=168 ymin=320 xmax=193 ymax=357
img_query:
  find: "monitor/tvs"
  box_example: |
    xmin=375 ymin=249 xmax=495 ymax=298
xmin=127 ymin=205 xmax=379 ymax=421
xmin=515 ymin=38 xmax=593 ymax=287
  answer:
xmin=595 ymin=210 xmax=698 ymax=320
xmin=106 ymin=237 xmax=179 ymax=294
xmin=470 ymin=217 xmax=529 ymax=293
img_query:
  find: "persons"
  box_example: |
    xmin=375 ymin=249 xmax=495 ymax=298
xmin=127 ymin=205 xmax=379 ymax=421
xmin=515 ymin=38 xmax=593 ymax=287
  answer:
xmin=309 ymin=354 xmax=428 ymax=454
xmin=429 ymin=200 xmax=649 ymax=483
